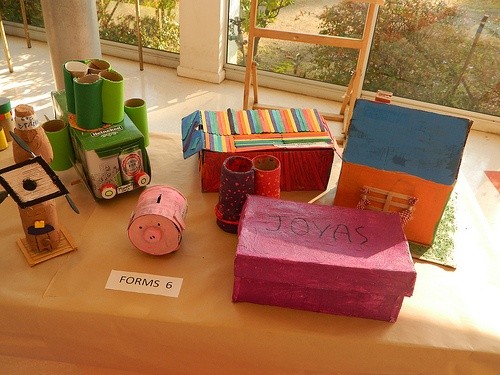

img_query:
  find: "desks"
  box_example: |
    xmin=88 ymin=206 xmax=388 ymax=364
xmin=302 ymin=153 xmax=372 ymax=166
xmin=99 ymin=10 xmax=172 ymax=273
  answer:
xmin=0 ymin=116 xmax=499 ymax=374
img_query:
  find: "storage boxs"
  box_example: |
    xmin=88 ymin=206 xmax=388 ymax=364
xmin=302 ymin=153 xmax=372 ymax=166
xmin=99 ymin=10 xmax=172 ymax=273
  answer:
xmin=182 ymin=108 xmax=344 ymax=193
xmin=230 ymin=195 xmax=417 ymax=322
